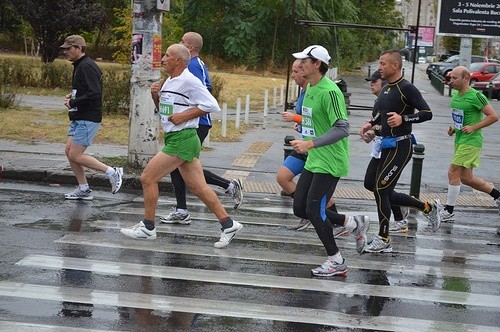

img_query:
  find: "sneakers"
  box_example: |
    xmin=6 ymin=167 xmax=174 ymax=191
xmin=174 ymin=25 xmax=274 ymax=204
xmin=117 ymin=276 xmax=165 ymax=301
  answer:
xmin=226 ymin=178 xmax=244 ymax=209
xmin=401 ymin=206 xmax=410 ymax=218
xmin=296 ymin=218 xmax=310 ymax=230
xmin=109 ymin=167 xmax=124 ymax=194
xmin=389 ymin=219 xmax=408 ymax=232
xmin=65 ymin=186 xmax=93 ymax=200
xmin=160 ymin=207 xmax=191 ymax=224
xmin=351 ymin=215 xmax=370 ymax=254
xmin=363 ymin=235 xmax=392 ymax=252
xmin=333 ymin=227 xmax=348 ymax=237
xmin=423 ymin=198 xmax=441 ymax=233
xmin=441 ymin=210 xmax=455 ymax=221
xmin=215 ymin=220 xmax=242 ymax=249
xmin=120 ymin=221 xmax=156 ymax=241
xmin=312 ymin=258 xmax=347 ymax=277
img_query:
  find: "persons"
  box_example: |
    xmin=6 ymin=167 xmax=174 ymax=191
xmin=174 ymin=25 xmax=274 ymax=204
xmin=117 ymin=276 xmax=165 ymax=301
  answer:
xmin=363 ymin=71 xmax=410 ymax=232
xmin=60 ymin=35 xmax=123 ymax=199
xmin=440 ymin=66 xmax=500 ymax=221
xmin=159 ymin=32 xmax=244 ymax=224
xmin=293 ymin=44 xmax=370 ymax=276
xmin=360 ymin=50 xmax=442 ymax=252
xmin=121 ymin=43 xmax=243 ymax=247
xmin=277 ymin=61 xmax=350 ymax=238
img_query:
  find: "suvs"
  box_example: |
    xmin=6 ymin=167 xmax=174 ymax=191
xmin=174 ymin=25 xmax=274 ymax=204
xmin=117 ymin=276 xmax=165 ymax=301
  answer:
xmin=426 ymin=54 xmax=500 ymax=88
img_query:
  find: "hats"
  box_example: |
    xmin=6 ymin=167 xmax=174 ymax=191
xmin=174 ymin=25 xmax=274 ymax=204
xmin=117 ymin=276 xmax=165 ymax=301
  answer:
xmin=292 ymin=45 xmax=330 ymax=66
xmin=60 ymin=35 xmax=86 ymax=48
xmin=365 ymin=70 xmax=382 ymax=82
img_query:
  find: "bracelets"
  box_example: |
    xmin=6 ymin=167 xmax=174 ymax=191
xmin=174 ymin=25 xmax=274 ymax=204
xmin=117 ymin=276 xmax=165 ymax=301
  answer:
xmin=294 ymin=114 xmax=302 ymax=123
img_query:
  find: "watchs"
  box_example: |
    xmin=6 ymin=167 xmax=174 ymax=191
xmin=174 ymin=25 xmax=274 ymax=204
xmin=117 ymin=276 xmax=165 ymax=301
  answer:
xmin=375 ymin=124 xmax=380 ymax=134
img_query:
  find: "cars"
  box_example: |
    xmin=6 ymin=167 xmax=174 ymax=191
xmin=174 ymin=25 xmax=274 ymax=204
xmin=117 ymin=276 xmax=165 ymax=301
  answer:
xmin=471 ymin=72 xmax=500 ymax=98
